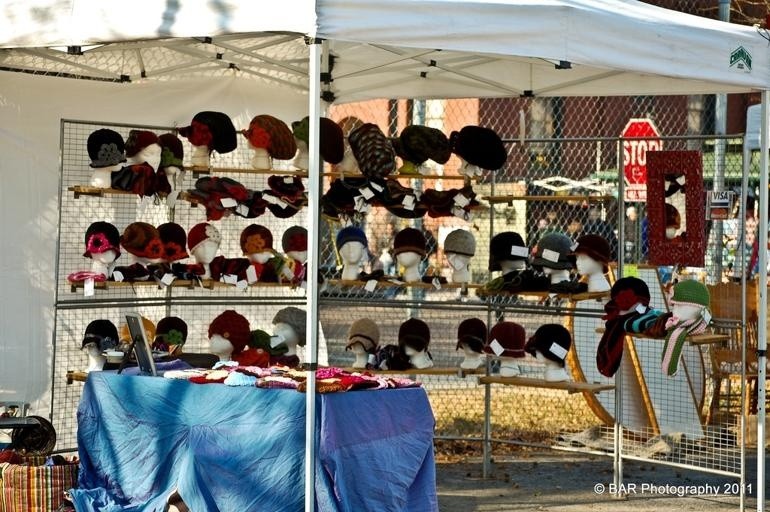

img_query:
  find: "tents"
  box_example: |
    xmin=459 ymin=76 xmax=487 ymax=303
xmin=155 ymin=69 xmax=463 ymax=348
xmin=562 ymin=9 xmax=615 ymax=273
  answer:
xmin=0 ymin=0 xmax=770 ymax=512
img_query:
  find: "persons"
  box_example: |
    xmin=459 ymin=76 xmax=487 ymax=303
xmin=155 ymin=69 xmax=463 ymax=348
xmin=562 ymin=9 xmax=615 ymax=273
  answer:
xmin=528 ymin=220 xmax=552 ymax=262
xmin=335 ymin=226 xmax=367 ymax=283
xmin=121 ymin=223 xmax=165 ymax=280
xmin=159 ymin=134 xmax=184 ymax=192
xmin=152 ymin=224 xmax=188 ymax=264
xmin=600 ymin=276 xmax=650 ymax=319
xmin=292 ymin=116 xmax=344 ymax=170
xmin=640 ymin=205 xmax=648 ymax=263
xmin=581 ymin=203 xmax=618 ymax=262
xmin=189 ymin=222 xmax=223 ymax=278
xmin=80 ymin=320 xmax=119 ymax=373
xmin=242 ymin=115 xmax=296 ymax=170
xmin=393 ymin=226 xmax=426 ymax=287
xmin=375 ymin=345 xmax=412 ymax=372
xmin=87 ymin=129 xmax=126 ymax=188
xmin=345 ymin=318 xmax=379 ymax=369
xmin=240 ymin=224 xmax=279 ymax=265
xmin=544 ymin=200 xmax=556 ymax=223
xmin=391 ymin=125 xmax=452 ymax=174
xmin=666 ymin=203 xmax=681 ymax=238
xmin=444 ymin=230 xmax=476 ymax=295
xmin=152 ymin=317 xmax=188 ymax=357
xmin=120 ymin=316 xmax=156 ymax=362
xmin=399 ymin=318 xmax=434 ymax=369
xmin=250 ymin=330 xmax=289 ymax=356
xmin=282 ymin=226 xmax=308 ymax=266
xmin=126 ymin=130 xmax=161 ymax=174
xmin=176 ymin=111 xmax=237 ymax=168
xmin=625 ymin=206 xmax=636 ymax=262
xmin=484 ymin=322 xmax=526 ymax=378
xmin=333 ymin=143 xmax=361 ymax=180
xmin=526 ymin=324 xmax=572 ymax=381
xmin=83 ymin=222 xmax=121 ymax=279
xmin=564 ymin=217 xmax=588 ymax=279
xmin=532 ymin=233 xmax=576 ymax=284
xmin=456 ymin=318 xmax=487 ymax=370
xmin=665 ymin=280 xmax=712 ymax=336
xmin=570 ymin=234 xmax=611 ymax=293
xmin=209 ymin=310 xmax=249 ymax=365
xmin=272 ymin=307 xmax=307 ymax=369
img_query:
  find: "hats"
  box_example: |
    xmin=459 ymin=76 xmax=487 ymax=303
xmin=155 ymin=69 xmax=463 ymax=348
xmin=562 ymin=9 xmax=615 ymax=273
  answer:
xmin=570 ymin=236 xmax=611 ymax=274
xmin=268 ymin=174 xmax=303 ymax=201
xmin=236 ymin=369 xmax=259 ymax=378
xmin=211 ymin=177 xmax=248 ymax=200
xmin=81 ymin=319 xmax=118 ymax=351
xmin=668 ymin=280 xmax=710 ymax=306
xmin=179 ymin=112 xmax=237 ymax=153
xmin=124 ymin=131 xmax=159 ymax=158
xmin=208 ymin=310 xmax=250 ymax=353
xmin=387 ymin=377 xmax=420 ymax=388
xmin=345 ymin=318 xmax=381 ymax=351
xmin=334 ymin=176 xmax=372 ymax=198
xmin=336 ymin=377 xmax=377 ymax=390
xmin=190 ymin=190 xmax=226 ymax=220
xmin=666 ymin=202 xmax=680 ymax=227
xmin=349 ymin=124 xmax=393 ymax=174
xmin=455 ymin=319 xmax=486 ymax=352
xmin=384 ymin=180 xmax=412 ymax=205
xmin=87 ymin=129 xmax=127 ymax=168
xmin=158 ymin=133 xmax=184 ymax=171
xmin=257 ymin=377 xmax=301 ymax=390
xmin=525 ymin=323 xmax=569 ymax=362
xmin=484 ymin=321 xmax=525 ymax=357
xmin=398 ymin=318 xmax=430 ymax=351
xmin=226 ymin=372 xmax=257 ymax=388
xmin=156 ymin=222 xmax=189 ymax=260
xmin=292 ymin=116 xmax=345 ymax=162
xmin=270 ymin=366 xmax=289 ymax=376
xmin=337 ymin=228 xmax=367 ymax=253
xmin=245 ymin=367 xmax=272 ymax=377
xmin=83 ymin=221 xmax=121 ymax=260
xmin=263 ymin=190 xmax=306 ymax=219
xmin=315 ymin=367 xmax=343 ymax=378
xmin=360 ymin=376 xmax=388 ymax=390
xmin=213 ymin=361 xmax=239 ymax=367
xmin=388 ymin=203 xmax=426 ymax=219
xmin=327 ymin=187 xmax=356 ymax=210
xmin=188 ymin=177 xmax=232 ymax=209
xmin=282 ymin=226 xmax=307 ymax=253
xmin=242 ymin=114 xmax=296 ymax=161
xmin=164 ymin=370 xmax=200 ymax=379
xmin=284 ymin=371 xmax=310 ymax=381
xmin=187 ymin=223 xmax=222 ymax=255
xmin=534 ymin=234 xmax=573 ymax=270
xmin=240 ymin=224 xmax=274 ymax=255
xmin=156 ymin=318 xmax=187 ymax=346
xmin=273 ymin=308 xmax=307 ymax=347
xmin=604 ymin=277 xmax=649 ymax=312
xmin=298 ymin=379 xmax=342 ymax=393
xmin=192 ymin=376 xmax=225 ymax=383
xmin=119 ymin=222 xmax=160 ymax=258
xmin=122 ymin=318 xmax=156 ymax=347
xmin=12 ymin=418 xmax=56 ymax=461
xmin=422 ymin=186 xmax=475 ymax=217
xmin=392 ymin=126 xmax=451 ymax=165
xmin=206 ymin=370 xmax=227 ymax=379
xmin=341 ymin=371 xmax=371 ymax=376
xmin=443 ymin=230 xmax=475 ymax=256
xmin=321 ymin=198 xmax=342 ymax=222
xmin=365 ymin=178 xmax=386 ymax=206
xmin=393 ymin=229 xmax=427 ymax=260
xmin=233 ymin=189 xmax=265 ymax=217
